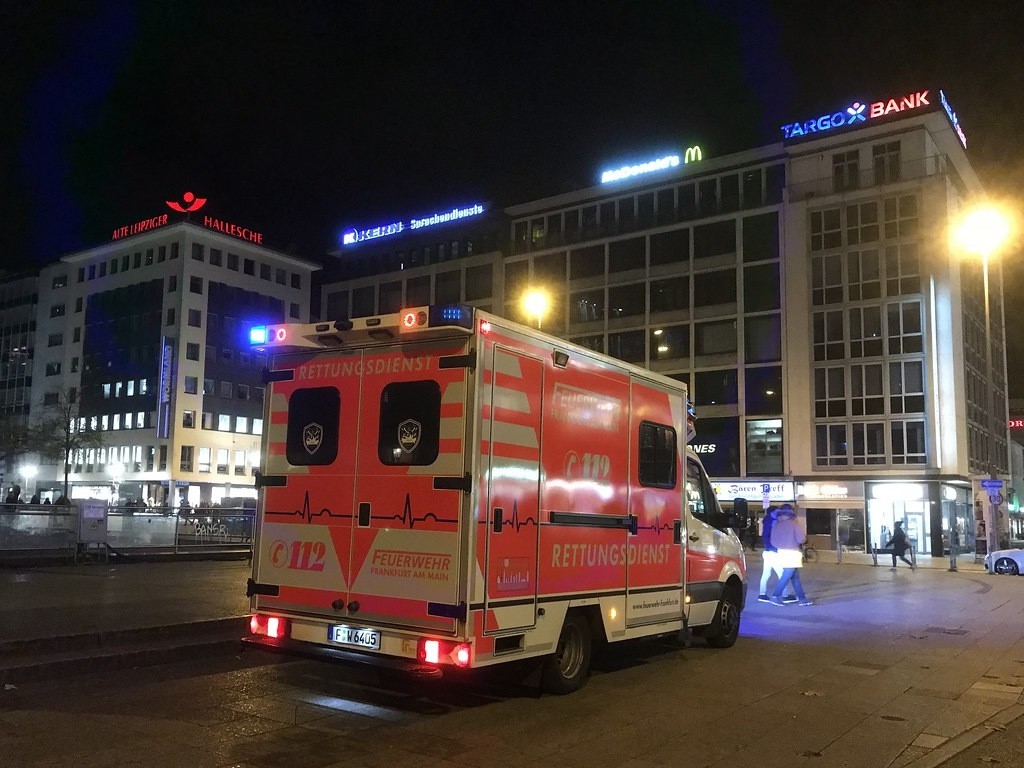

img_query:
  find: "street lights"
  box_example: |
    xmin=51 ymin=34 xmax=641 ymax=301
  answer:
xmin=952 ymin=205 xmax=1018 ymax=482
xmin=21 ymin=464 xmax=35 ymax=491
xmin=108 ymin=461 xmax=123 ymax=507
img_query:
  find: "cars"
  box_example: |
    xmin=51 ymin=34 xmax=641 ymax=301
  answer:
xmin=983 ymin=546 xmax=1024 ymax=575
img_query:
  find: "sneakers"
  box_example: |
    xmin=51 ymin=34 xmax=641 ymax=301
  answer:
xmin=770 ymin=597 xmax=785 ymax=606
xmin=757 ymin=594 xmax=770 ymax=602
xmin=799 ymin=599 xmax=813 ymax=606
xmin=908 ymin=566 xmax=918 ymax=569
xmin=889 ymin=568 xmax=897 ymax=572
xmin=782 ymin=595 xmax=799 ymax=603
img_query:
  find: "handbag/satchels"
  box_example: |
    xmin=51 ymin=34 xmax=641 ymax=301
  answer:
xmin=904 ymin=537 xmax=912 ymax=549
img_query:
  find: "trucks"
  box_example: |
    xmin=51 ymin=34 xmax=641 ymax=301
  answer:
xmin=242 ymin=306 xmax=752 ymax=699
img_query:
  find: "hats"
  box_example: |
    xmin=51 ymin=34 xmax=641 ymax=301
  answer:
xmin=895 ymin=521 xmax=903 ymax=527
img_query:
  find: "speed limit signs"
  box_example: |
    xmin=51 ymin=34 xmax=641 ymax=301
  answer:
xmin=990 ymin=493 xmax=1004 ymax=505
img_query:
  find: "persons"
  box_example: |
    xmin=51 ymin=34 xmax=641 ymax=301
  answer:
xmin=886 ymin=521 xmax=913 ymax=570
xmin=1000 ymin=533 xmax=1014 ymax=549
xmin=769 ymin=504 xmax=812 ymax=607
xmin=950 ymin=525 xmax=960 ymax=570
xmin=125 ymin=498 xmax=168 ymax=512
xmin=758 ymin=507 xmax=798 ymax=603
xmin=5 ymin=484 xmax=71 ymax=505
xmin=748 ymin=516 xmax=758 ymax=551
xmin=183 ymin=501 xmax=193 ymax=526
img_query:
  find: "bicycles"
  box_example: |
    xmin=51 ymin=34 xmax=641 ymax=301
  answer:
xmin=800 ymin=542 xmax=818 ymax=563
